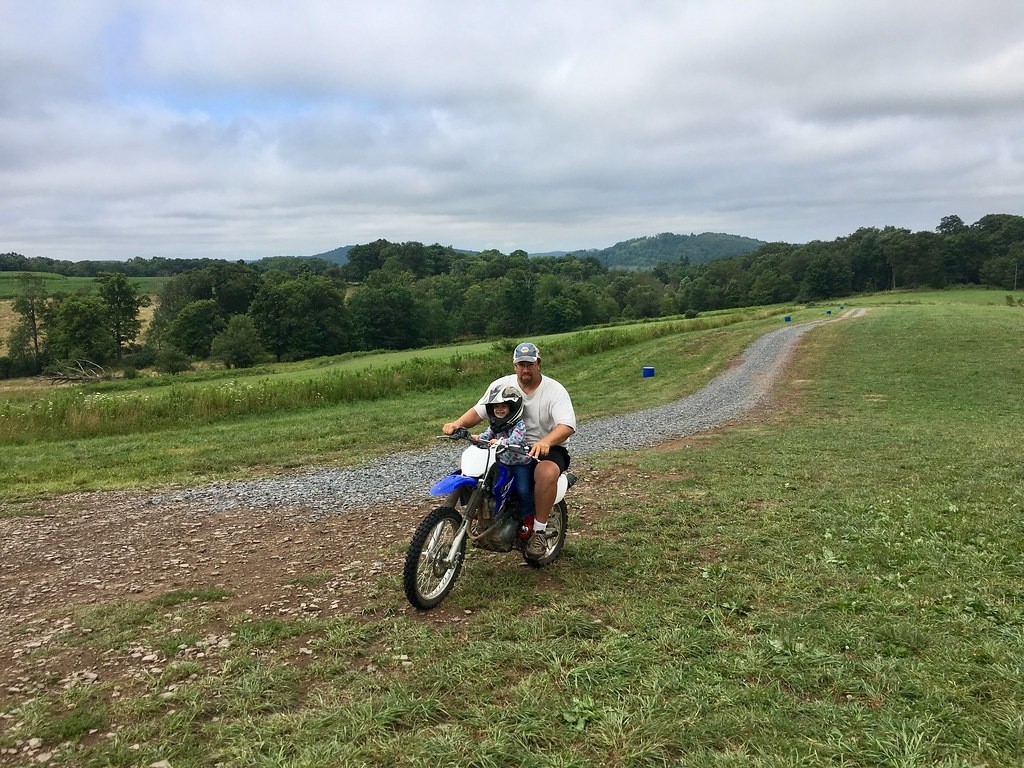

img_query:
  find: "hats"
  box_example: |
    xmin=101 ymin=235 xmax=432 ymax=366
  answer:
xmin=513 ymin=343 xmax=540 ymax=363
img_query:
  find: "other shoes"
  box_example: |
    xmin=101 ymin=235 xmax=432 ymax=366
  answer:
xmin=473 ymin=514 xmax=478 ymax=520
xmin=519 ymin=514 xmax=533 ymax=538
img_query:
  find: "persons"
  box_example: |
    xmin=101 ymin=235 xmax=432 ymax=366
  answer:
xmin=470 ymin=385 xmax=535 ymax=539
xmin=442 ymin=343 xmax=576 ymax=559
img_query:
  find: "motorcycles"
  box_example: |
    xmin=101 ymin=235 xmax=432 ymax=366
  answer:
xmin=403 ymin=425 xmax=580 ymax=611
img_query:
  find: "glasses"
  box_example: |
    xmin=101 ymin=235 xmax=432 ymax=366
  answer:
xmin=515 ymin=363 xmax=538 ymax=370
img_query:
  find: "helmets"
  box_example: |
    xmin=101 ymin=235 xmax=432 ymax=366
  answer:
xmin=480 ymin=385 xmax=524 ymax=433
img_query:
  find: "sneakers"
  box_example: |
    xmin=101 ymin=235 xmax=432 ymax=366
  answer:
xmin=527 ymin=530 xmax=547 ymax=557
